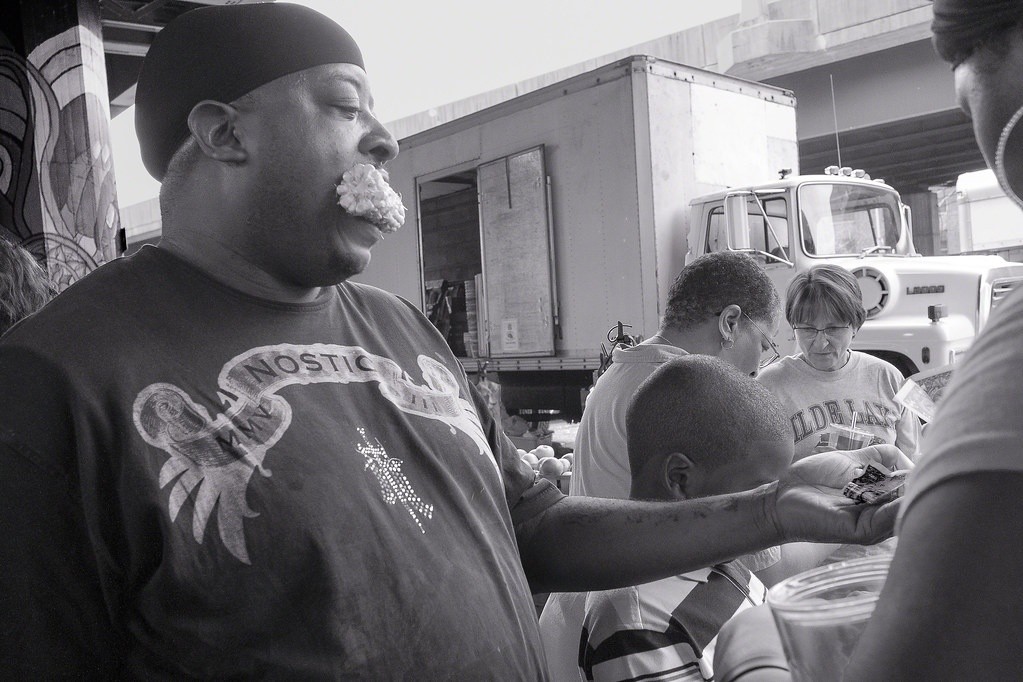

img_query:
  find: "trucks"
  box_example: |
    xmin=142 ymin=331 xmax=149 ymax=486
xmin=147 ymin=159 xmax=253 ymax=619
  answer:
xmin=121 ymin=55 xmax=1023 ymax=446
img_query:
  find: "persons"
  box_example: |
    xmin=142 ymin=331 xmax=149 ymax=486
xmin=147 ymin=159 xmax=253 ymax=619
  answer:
xmin=841 ymin=0 xmax=1023 ymax=682
xmin=0 ymin=3 xmax=922 ymax=682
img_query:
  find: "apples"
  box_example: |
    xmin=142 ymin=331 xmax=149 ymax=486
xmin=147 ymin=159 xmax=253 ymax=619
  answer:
xmin=515 ymin=445 xmax=572 ymax=477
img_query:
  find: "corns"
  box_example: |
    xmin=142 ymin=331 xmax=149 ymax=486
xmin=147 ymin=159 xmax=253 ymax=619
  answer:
xmin=335 ymin=164 xmax=405 ymax=233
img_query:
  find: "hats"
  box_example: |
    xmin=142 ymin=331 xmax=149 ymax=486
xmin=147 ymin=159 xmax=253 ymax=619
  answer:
xmin=931 ymin=0 xmax=1023 ymax=70
xmin=134 ymin=2 xmax=367 ymax=184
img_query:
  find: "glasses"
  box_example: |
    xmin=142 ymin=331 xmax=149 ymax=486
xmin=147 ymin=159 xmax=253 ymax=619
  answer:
xmin=714 ymin=308 xmax=781 ymax=370
xmin=792 ymin=321 xmax=853 ymax=341
